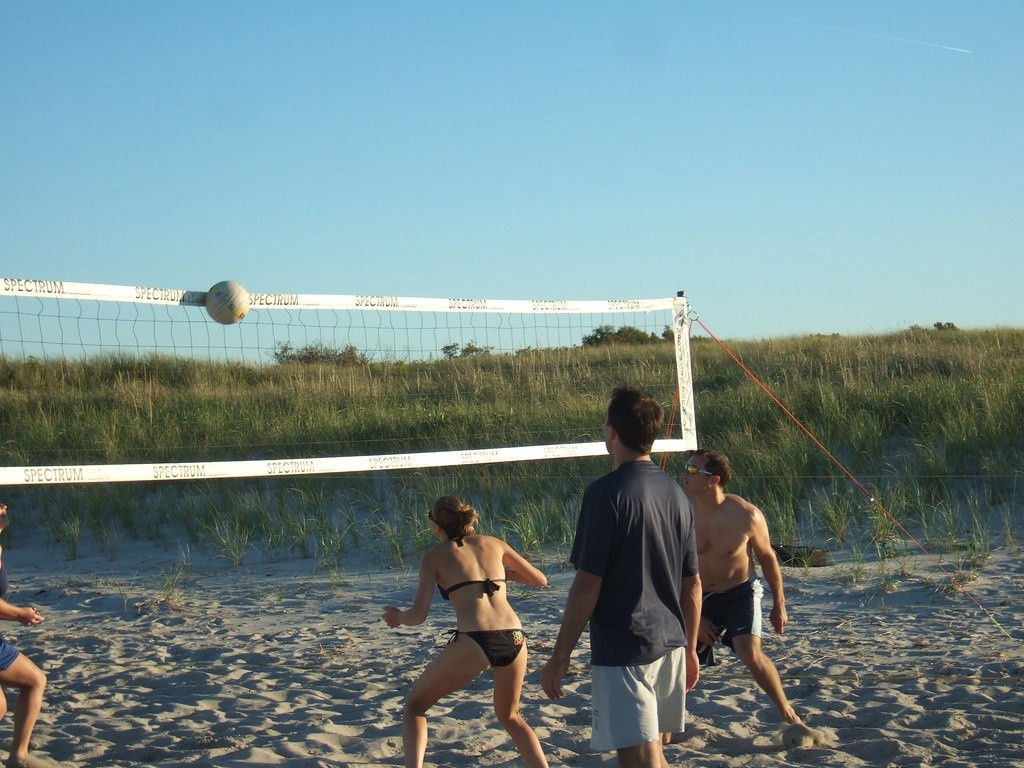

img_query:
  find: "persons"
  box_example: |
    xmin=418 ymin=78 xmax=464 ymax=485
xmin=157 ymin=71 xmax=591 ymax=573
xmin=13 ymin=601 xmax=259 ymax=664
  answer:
xmin=540 ymin=386 xmax=702 ymax=768
xmin=664 ymin=449 xmax=802 ymax=743
xmin=383 ymin=495 xmax=549 ymax=768
xmin=0 ymin=503 xmax=48 ymax=760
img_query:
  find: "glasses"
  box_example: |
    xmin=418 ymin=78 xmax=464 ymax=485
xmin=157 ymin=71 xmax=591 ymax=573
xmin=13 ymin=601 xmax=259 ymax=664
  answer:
xmin=429 ymin=510 xmax=440 ymax=525
xmin=684 ymin=464 xmax=717 ymax=475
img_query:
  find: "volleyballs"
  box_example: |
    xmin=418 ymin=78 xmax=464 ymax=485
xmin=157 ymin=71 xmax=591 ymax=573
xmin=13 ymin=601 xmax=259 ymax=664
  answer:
xmin=205 ymin=281 xmax=250 ymax=326
